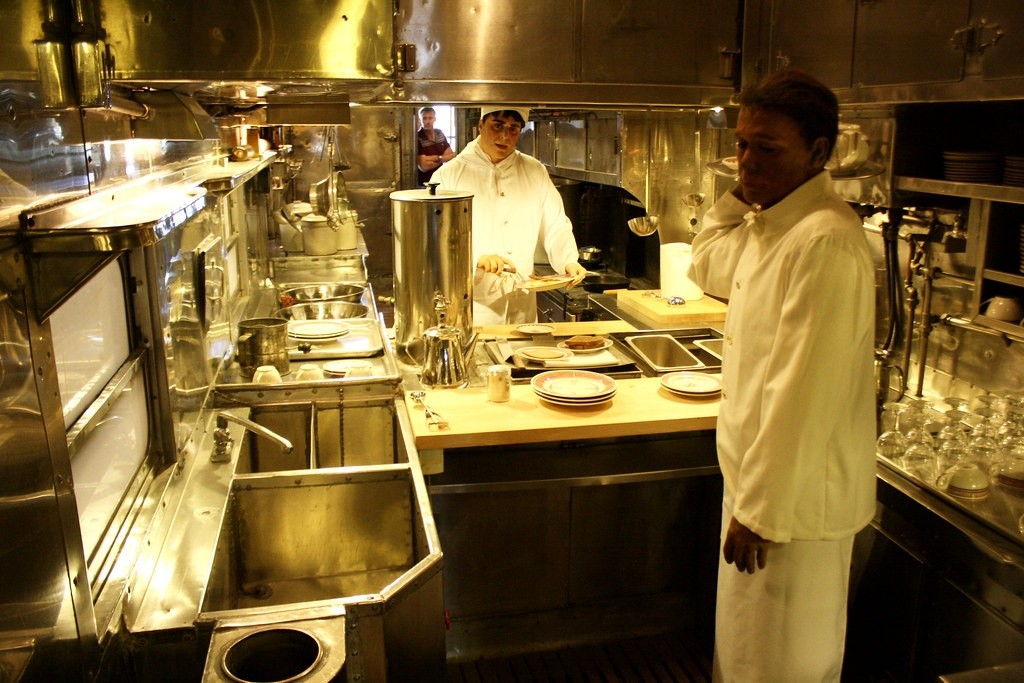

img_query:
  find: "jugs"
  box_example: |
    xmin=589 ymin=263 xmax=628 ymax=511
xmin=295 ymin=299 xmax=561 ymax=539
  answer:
xmin=236 ymin=317 xmax=292 ymax=379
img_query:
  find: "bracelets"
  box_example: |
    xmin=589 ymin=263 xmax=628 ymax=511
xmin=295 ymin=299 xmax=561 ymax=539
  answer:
xmin=438 ymin=155 xmax=442 ymax=162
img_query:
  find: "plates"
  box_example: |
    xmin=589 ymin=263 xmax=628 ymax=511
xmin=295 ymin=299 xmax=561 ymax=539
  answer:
xmin=530 ymin=369 xmax=617 ymax=406
xmin=287 ymin=319 xmax=351 ymax=344
xmin=322 ymin=360 xmax=372 ymax=375
xmin=556 ymin=338 xmax=613 ymax=354
xmin=515 ymin=346 xmax=574 ymax=361
xmin=938 ymin=146 xmax=1024 ymax=186
xmin=515 ymin=276 xmax=575 ymax=292
xmin=516 ymin=323 xmax=556 ymax=334
xmin=660 ymin=372 xmax=722 ymax=397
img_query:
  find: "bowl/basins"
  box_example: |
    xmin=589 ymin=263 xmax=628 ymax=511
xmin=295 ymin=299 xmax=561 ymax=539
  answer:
xmin=296 ymin=364 xmax=324 ymax=381
xmin=271 ymin=301 xmax=370 ymax=322
xmin=277 ymin=284 xmax=366 ymax=309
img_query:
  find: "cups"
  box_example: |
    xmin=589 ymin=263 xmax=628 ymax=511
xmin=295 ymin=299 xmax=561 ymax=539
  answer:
xmin=935 ymin=463 xmax=989 ymax=500
xmin=486 ymin=364 xmax=511 ymax=403
xmin=251 ymin=366 xmax=282 ymax=384
xmin=979 ymin=295 xmax=1022 ymax=322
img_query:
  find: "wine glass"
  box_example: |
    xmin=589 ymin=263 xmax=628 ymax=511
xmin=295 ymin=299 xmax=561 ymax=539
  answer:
xmin=875 ymin=394 xmax=1024 ymax=485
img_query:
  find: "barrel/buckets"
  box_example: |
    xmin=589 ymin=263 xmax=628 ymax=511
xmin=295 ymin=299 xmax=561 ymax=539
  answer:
xmin=388 ymin=182 xmax=474 ymax=367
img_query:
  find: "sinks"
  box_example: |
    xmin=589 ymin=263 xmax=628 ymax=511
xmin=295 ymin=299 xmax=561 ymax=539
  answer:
xmin=199 ymin=467 xmax=430 ymax=613
xmin=234 ymin=397 xmax=412 ymax=474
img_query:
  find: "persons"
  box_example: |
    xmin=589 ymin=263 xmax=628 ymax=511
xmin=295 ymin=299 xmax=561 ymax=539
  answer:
xmin=426 ymin=106 xmax=587 ymax=325
xmin=416 ymin=107 xmax=456 ymax=187
xmin=686 ymin=72 xmax=877 ymax=683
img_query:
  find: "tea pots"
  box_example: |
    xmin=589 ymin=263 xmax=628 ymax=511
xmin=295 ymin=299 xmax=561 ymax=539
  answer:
xmin=405 ymin=294 xmax=480 ymax=391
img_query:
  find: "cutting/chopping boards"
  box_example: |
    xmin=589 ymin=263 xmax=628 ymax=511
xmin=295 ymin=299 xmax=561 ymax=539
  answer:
xmin=616 ymin=288 xmax=728 ymax=324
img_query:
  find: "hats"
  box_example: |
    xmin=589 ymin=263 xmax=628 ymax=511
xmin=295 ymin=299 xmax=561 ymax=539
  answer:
xmin=480 ymin=105 xmax=530 ymax=123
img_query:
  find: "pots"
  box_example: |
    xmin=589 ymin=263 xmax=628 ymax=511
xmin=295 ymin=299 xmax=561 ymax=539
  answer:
xmin=278 ymin=200 xmax=359 ymax=256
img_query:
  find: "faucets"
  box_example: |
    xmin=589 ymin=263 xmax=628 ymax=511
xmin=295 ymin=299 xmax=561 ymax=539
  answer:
xmin=209 ymin=412 xmax=294 ymax=463
xmin=432 ymin=295 xmax=451 ymax=329
